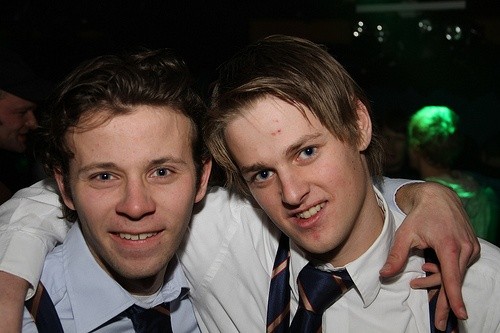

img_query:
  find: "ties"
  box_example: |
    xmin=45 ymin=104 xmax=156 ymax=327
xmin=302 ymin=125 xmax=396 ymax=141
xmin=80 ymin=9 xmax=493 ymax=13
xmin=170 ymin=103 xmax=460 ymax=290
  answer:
xmin=286 ymin=264 xmax=353 ymax=333
xmin=123 ymin=302 xmax=173 ymax=333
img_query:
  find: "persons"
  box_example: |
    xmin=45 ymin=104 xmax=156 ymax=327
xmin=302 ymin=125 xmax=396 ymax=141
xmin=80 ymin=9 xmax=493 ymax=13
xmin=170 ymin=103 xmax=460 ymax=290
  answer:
xmin=0 ymin=35 xmax=500 ymax=333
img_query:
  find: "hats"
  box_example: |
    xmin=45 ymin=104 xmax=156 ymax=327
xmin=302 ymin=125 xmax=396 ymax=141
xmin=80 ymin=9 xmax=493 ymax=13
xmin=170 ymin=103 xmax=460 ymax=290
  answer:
xmin=0 ymin=72 xmax=42 ymax=104
